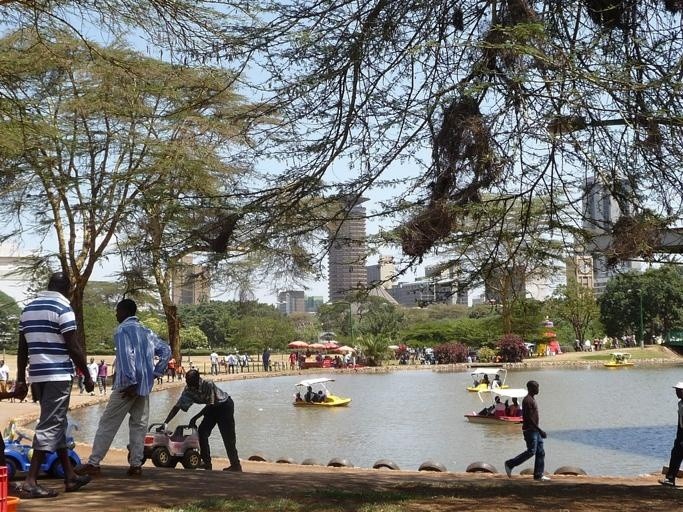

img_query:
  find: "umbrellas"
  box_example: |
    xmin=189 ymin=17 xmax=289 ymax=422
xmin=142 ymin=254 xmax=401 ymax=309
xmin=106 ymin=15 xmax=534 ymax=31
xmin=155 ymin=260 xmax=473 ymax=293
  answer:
xmin=309 ymin=343 xmax=323 ymax=361
xmin=323 ymin=343 xmax=339 ymax=356
xmin=336 ymin=345 xmax=355 ymax=356
xmin=288 ymin=341 xmax=308 ymax=368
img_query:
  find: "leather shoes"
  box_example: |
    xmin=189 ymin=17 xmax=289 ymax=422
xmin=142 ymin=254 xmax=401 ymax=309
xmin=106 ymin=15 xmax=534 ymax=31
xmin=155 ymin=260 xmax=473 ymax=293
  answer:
xmin=127 ymin=466 xmax=141 ymax=477
xmin=17 ymin=484 xmax=57 ymax=498
xmin=65 ymin=474 xmax=89 ymax=491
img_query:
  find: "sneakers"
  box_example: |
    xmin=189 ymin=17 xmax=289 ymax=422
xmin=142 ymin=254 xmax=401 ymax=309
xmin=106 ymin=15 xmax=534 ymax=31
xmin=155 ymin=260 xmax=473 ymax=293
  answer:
xmin=505 ymin=462 xmax=511 ymax=478
xmin=74 ymin=464 xmax=100 ymax=474
xmin=659 ymin=479 xmax=673 ymax=485
xmin=534 ymin=475 xmax=550 ymax=481
xmin=224 ymin=464 xmax=242 ymax=472
xmin=199 ymin=460 xmax=212 ymax=469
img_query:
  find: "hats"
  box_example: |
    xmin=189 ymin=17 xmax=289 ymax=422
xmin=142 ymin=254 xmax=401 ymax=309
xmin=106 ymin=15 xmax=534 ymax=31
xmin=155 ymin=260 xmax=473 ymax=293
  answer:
xmin=673 ymin=382 xmax=683 ymax=389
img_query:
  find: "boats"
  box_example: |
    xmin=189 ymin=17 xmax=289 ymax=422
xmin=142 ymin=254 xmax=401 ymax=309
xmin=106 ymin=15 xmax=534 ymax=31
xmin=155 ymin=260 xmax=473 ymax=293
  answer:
xmin=465 ymin=367 xmax=511 ymax=392
xmin=293 ymin=377 xmax=353 ymax=408
xmin=463 ymin=387 xmax=531 ymax=427
xmin=604 ymin=352 xmax=634 ymax=368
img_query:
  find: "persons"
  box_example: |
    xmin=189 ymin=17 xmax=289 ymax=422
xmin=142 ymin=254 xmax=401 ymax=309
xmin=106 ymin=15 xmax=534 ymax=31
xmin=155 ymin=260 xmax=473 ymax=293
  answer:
xmin=658 ymin=381 xmax=683 ymax=486
xmin=575 ymin=333 xmax=636 ymax=352
xmin=289 ymin=348 xmax=367 ymax=371
xmin=464 ymin=354 xmax=476 ymax=363
xmin=296 ymin=386 xmax=325 ymax=403
xmin=157 ymin=370 xmax=242 ymax=472
xmin=481 ymin=374 xmax=490 ymax=387
xmin=70 ymin=348 xmax=117 ymax=395
xmin=262 ymin=350 xmax=272 ymax=371
xmin=210 ymin=350 xmax=248 ymax=375
xmin=14 ymin=272 xmax=94 ymax=499
xmin=78 ymin=299 xmax=172 ymax=476
xmin=153 ymin=356 xmax=199 ymax=384
xmin=509 ymin=397 xmax=520 ymax=416
xmin=504 ymin=381 xmax=550 ymax=480
xmin=396 ymin=344 xmax=437 ymax=365
xmin=492 ymin=352 xmax=504 ymax=363
xmin=0 ymin=391 xmax=31 ymax=499
xmin=491 ymin=375 xmax=501 ymax=387
xmin=0 ymin=356 xmax=36 ymax=403
xmin=491 ymin=396 xmax=506 ymax=416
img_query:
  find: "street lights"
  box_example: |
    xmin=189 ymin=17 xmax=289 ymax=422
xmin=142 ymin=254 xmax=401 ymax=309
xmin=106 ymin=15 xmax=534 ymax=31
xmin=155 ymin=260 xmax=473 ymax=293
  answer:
xmin=627 ymin=287 xmax=644 ymax=346
xmin=326 ymin=302 xmax=354 ymax=350
